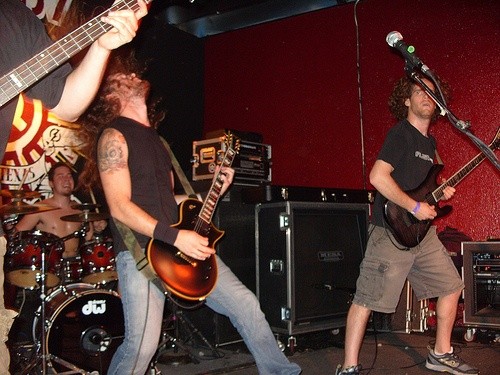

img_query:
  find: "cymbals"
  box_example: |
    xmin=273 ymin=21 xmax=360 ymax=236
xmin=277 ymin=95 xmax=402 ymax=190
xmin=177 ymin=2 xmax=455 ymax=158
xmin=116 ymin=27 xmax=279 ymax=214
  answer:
xmin=0 ymin=189 xmax=43 ymax=199
xmin=0 ymin=204 xmax=64 ymax=216
xmin=70 ymin=203 xmax=103 ymax=210
xmin=59 ymin=212 xmax=110 ymax=222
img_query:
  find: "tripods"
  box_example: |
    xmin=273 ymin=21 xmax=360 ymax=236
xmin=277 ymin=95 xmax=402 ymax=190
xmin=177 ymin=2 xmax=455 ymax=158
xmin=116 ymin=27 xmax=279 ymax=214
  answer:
xmin=19 ymin=222 xmax=91 ymax=375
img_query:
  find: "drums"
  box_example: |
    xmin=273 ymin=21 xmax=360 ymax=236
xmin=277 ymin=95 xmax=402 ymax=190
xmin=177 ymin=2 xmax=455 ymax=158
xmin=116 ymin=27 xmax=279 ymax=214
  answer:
xmin=78 ymin=237 xmax=122 ymax=285
xmin=60 ymin=255 xmax=83 ymax=284
xmin=32 ymin=282 xmax=161 ymax=375
xmin=2 ymin=227 xmax=66 ymax=291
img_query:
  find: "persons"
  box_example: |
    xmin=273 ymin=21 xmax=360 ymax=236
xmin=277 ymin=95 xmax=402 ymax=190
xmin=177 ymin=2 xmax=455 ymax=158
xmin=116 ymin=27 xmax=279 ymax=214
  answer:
xmin=77 ymin=50 xmax=301 ymax=375
xmin=0 ymin=0 xmax=148 ymax=375
xmin=336 ymin=74 xmax=480 ymax=375
xmin=0 ymin=163 xmax=107 ymax=256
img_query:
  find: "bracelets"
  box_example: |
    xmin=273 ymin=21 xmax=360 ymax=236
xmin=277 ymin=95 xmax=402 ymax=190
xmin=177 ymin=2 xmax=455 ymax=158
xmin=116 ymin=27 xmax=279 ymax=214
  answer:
xmin=411 ymin=202 xmax=420 ymax=214
xmin=153 ymin=221 xmax=179 ymax=244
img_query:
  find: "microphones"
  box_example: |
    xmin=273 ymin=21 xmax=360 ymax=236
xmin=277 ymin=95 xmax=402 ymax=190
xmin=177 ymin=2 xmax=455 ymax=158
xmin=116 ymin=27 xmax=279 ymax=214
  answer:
xmin=386 ymin=30 xmax=431 ymax=76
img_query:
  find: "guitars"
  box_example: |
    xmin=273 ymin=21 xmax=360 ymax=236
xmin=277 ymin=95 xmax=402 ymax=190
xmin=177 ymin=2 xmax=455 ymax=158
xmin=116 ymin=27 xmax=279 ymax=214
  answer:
xmin=144 ymin=131 xmax=242 ymax=303
xmin=383 ymin=127 xmax=500 ymax=248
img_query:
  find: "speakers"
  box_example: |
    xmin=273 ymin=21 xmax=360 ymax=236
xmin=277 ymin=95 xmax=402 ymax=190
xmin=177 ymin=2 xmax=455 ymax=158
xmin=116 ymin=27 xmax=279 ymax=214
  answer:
xmin=294 ymin=209 xmax=364 ymax=321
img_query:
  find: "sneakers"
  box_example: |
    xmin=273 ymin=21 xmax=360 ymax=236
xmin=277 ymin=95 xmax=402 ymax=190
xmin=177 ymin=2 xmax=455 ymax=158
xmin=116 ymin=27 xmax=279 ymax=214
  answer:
xmin=335 ymin=363 xmax=361 ymax=375
xmin=425 ymin=345 xmax=481 ymax=375
